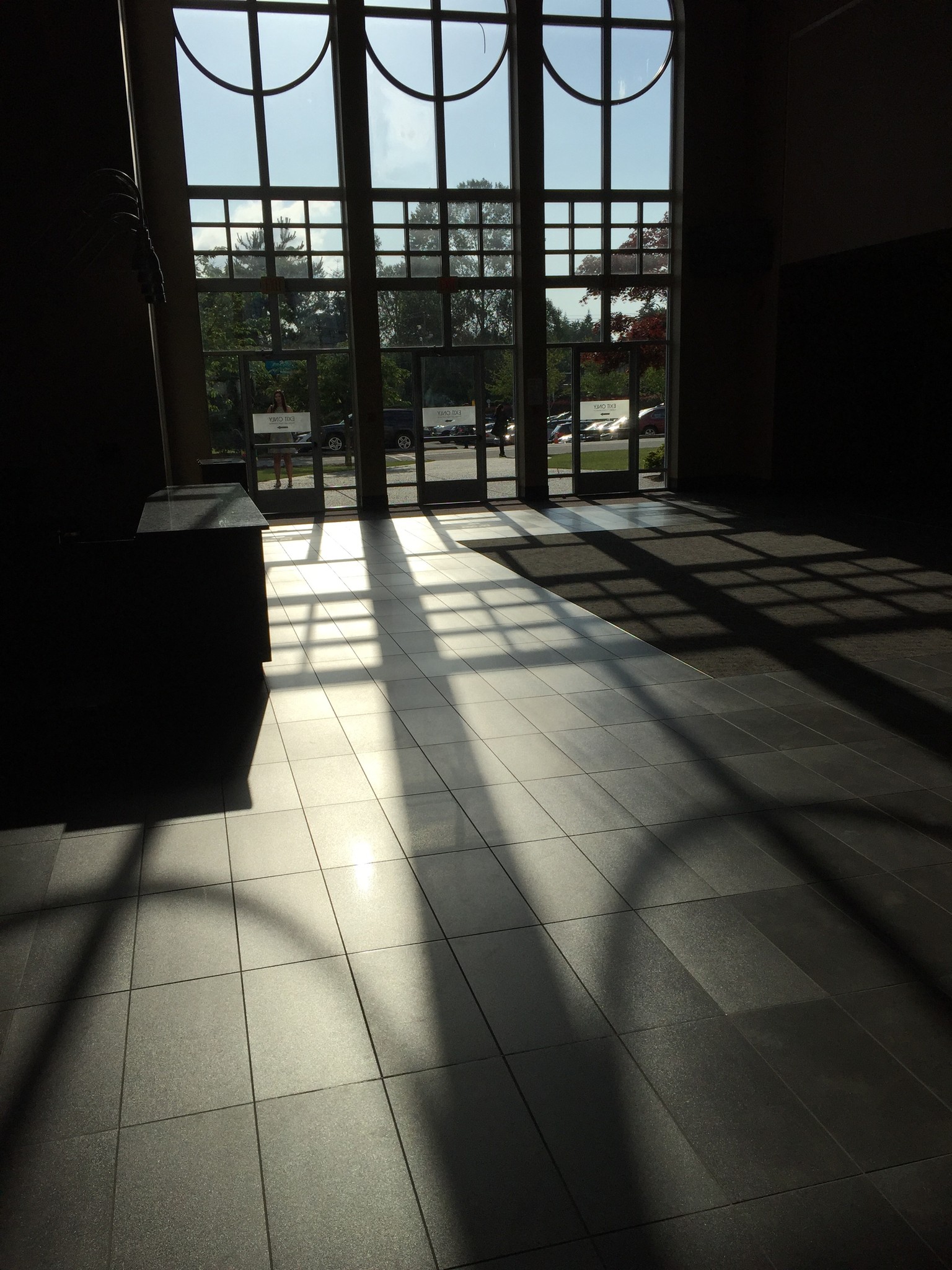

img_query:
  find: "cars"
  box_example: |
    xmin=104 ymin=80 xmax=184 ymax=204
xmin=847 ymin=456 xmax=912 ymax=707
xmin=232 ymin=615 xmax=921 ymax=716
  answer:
xmin=432 ymin=403 xmax=666 ymax=444
xmin=295 ymin=433 xmax=311 ymax=442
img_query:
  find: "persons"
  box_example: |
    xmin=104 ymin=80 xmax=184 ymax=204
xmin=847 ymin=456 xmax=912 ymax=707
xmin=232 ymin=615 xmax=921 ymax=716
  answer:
xmin=504 ymin=402 xmax=513 ymax=422
xmin=461 ymin=402 xmax=477 ymax=449
xmin=490 ymin=404 xmax=509 ymax=457
xmin=262 ymin=390 xmax=298 ymax=487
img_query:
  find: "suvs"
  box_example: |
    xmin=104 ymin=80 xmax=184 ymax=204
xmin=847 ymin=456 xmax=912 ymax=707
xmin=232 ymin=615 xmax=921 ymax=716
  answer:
xmin=320 ymin=409 xmax=414 ymax=451
